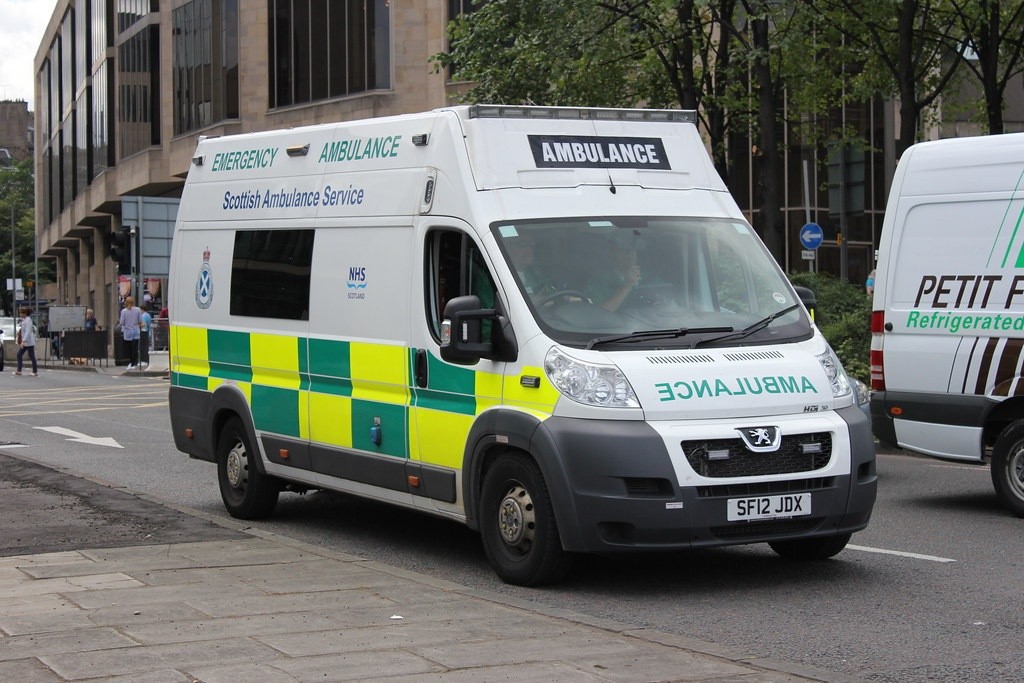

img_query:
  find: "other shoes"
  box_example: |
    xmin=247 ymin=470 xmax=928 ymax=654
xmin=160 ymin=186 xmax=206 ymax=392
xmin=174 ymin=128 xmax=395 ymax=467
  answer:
xmin=137 ymin=361 xmax=148 ymax=366
xmin=29 ymin=372 xmax=38 ymax=376
xmin=126 ymin=363 xmax=136 ymax=370
xmin=12 ymin=371 xmax=21 ymax=375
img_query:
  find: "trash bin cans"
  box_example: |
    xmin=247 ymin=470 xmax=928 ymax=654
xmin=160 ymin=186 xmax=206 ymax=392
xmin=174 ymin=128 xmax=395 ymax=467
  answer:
xmin=114 ymin=328 xmax=132 ymax=366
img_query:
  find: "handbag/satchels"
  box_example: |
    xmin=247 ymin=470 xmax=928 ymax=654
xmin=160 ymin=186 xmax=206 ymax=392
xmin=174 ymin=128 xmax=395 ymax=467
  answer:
xmin=17 ymin=329 xmax=22 ymax=344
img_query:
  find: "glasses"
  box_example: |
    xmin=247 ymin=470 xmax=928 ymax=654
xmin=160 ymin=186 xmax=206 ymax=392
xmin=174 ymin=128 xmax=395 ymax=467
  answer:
xmin=507 ymin=239 xmax=535 ymax=248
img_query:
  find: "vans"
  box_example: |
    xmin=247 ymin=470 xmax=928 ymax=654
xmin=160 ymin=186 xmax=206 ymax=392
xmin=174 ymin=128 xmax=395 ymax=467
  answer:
xmin=865 ymin=130 xmax=1024 ymax=512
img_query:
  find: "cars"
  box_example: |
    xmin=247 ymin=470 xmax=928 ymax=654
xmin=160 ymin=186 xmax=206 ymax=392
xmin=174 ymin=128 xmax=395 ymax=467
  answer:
xmin=0 ymin=317 xmax=39 ymax=339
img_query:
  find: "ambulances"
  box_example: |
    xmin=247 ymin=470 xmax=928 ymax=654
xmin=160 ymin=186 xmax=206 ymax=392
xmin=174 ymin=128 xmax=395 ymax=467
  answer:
xmin=165 ymin=103 xmax=881 ymax=583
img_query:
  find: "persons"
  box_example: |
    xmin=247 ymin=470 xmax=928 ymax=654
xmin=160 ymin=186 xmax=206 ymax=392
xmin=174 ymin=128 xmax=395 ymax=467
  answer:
xmin=117 ymin=296 xmax=143 ymax=369
xmin=86 ymin=309 xmax=97 ymax=331
xmin=96 ymin=325 xmax=101 ymax=331
xmin=865 ymin=270 xmax=876 ymax=300
xmin=157 ymin=307 xmax=168 ymax=346
xmin=587 ymin=232 xmax=682 ymax=314
xmin=138 ymin=305 xmax=152 ymax=366
xmin=11 ymin=305 xmax=39 ymax=377
xmin=469 ymin=226 xmax=594 ymax=343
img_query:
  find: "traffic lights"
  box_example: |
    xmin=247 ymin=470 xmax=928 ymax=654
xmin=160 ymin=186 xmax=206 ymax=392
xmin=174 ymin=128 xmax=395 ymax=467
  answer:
xmin=110 ymin=225 xmax=131 ymax=268
